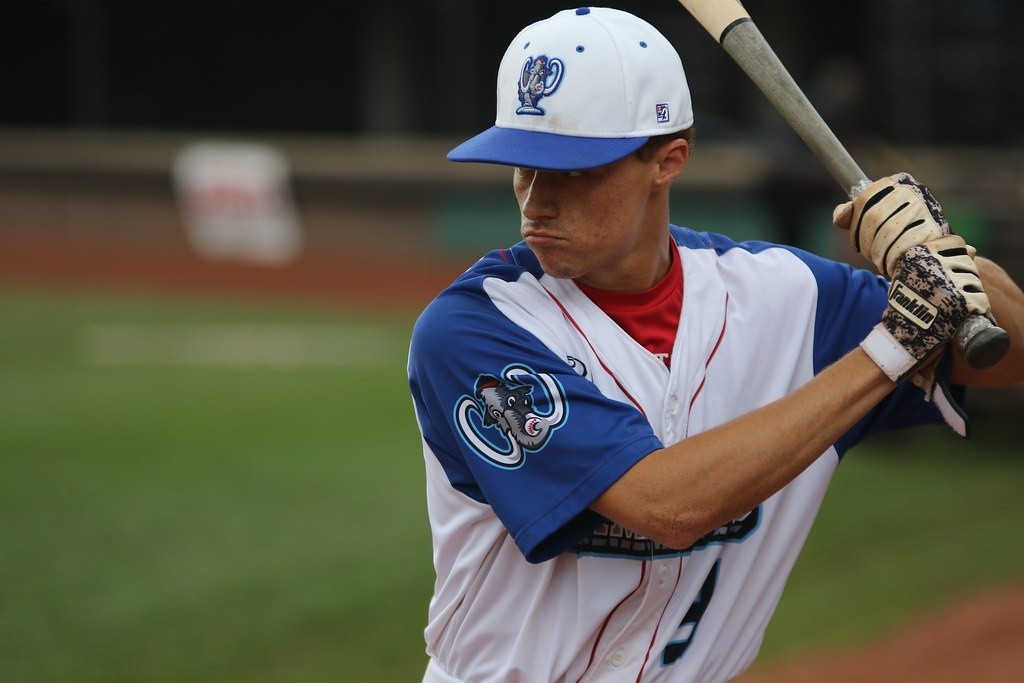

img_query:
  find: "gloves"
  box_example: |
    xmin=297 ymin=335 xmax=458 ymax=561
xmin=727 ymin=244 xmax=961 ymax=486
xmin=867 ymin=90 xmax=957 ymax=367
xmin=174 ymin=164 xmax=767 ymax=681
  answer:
xmin=832 ymin=172 xmax=949 ymax=279
xmin=859 ymin=234 xmax=993 ymax=438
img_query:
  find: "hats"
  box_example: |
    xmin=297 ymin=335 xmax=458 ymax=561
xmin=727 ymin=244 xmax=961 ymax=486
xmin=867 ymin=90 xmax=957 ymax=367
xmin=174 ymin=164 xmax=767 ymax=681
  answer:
xmin=448 ymin=6 xmax=694 ymax=172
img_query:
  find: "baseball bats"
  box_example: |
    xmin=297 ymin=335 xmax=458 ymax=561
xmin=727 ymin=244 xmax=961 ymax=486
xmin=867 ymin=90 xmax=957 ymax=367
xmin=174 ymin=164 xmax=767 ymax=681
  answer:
xmin=677 ymin=1 xmax=1010 ymax=371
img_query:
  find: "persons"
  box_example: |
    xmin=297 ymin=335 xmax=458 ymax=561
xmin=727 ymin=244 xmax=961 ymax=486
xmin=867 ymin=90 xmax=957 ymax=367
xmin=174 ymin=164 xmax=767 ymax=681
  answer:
xmin=407 ymin=7 xmax=1024 ymax=683
